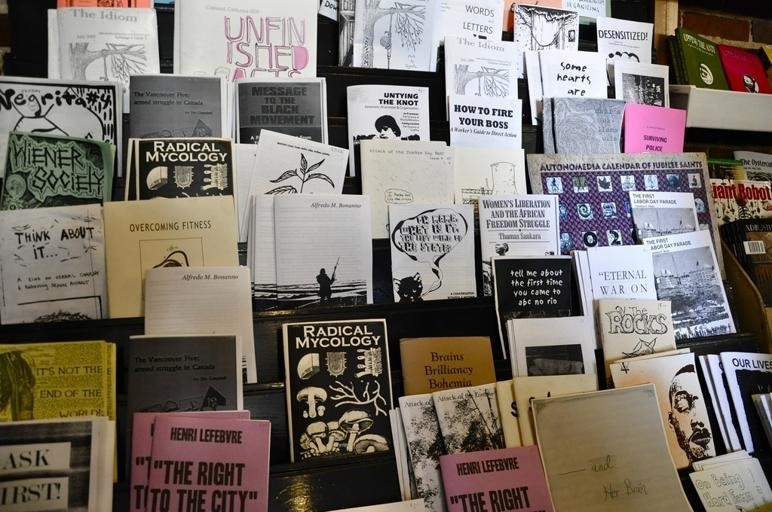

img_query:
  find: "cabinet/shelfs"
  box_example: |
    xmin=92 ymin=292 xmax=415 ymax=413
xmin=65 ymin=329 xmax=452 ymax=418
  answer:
xmin=0 ymin=6 xmax=757 ymax=512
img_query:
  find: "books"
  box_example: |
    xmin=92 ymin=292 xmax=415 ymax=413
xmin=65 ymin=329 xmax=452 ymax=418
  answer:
xmin=0 ymin=0 xmax=772 ymax=511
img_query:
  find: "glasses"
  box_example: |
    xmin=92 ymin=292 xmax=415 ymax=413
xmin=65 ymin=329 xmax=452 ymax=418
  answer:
xmin=377 ymin=125 xmax=390 ymax=133
